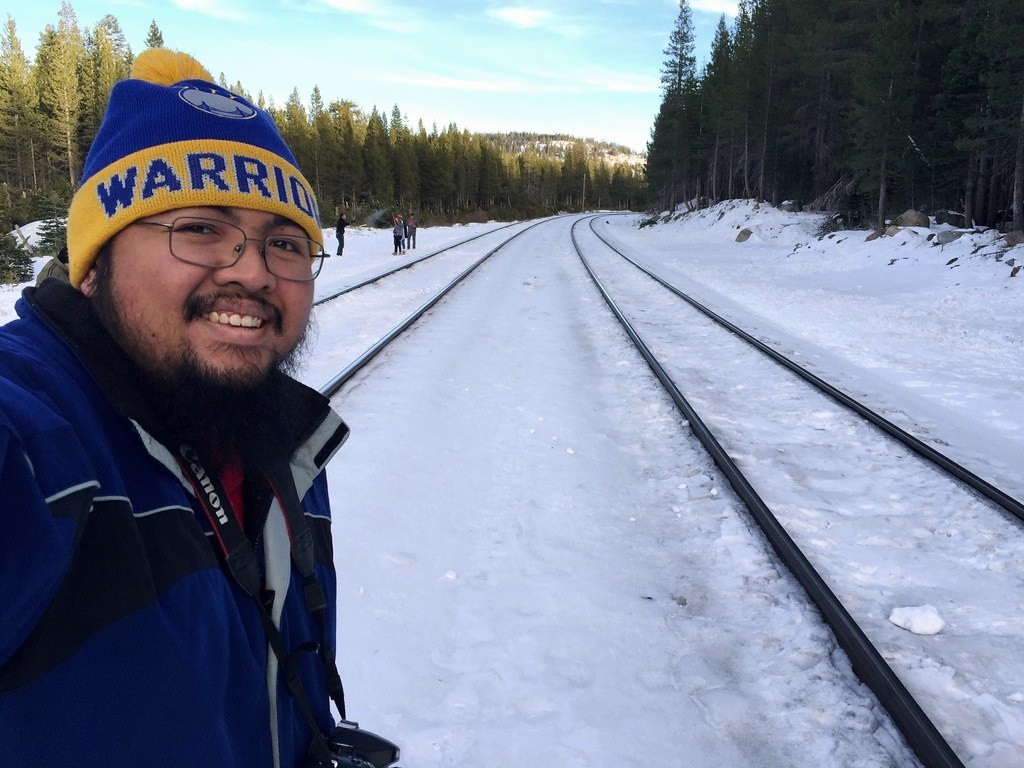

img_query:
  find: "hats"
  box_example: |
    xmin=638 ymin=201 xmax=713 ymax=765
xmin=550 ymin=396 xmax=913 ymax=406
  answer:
xmin=69 ymin=48 xmax=322 ymax=285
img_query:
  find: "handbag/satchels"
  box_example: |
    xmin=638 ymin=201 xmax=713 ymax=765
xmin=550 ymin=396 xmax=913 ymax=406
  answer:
xmin=306 ymin=720 xmax=400 ymax=768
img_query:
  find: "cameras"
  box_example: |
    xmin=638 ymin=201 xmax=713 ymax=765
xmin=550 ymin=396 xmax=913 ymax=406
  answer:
xmin=316 ymin=719 xmax=400 ymax=768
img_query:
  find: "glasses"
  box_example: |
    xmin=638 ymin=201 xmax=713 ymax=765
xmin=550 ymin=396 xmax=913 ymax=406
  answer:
xmin=134 ymin=217 xmax=330 ymax=283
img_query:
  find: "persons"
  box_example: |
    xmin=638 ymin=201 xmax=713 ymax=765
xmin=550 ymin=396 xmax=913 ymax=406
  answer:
xmin=336 ymin=213 xmax=355 ymax=256
xmin=406 ymin=213 xmax=419 ymax=250
xmin=0 ymin=48 xmax=351 ymax=768
xmin=392 ymin=215 xmax=408 ymax=256
